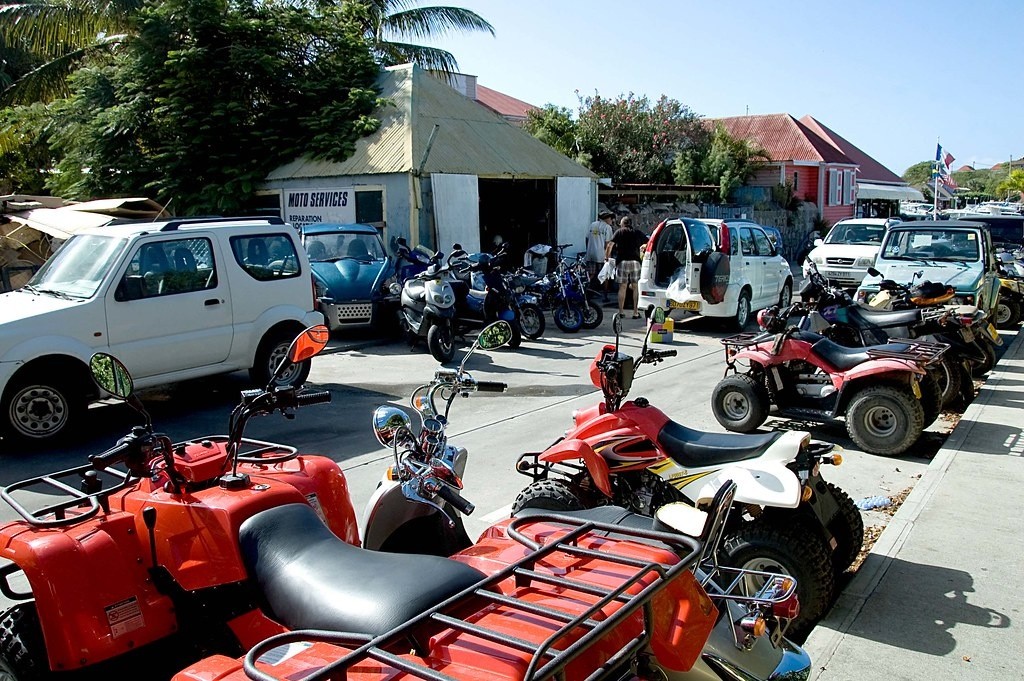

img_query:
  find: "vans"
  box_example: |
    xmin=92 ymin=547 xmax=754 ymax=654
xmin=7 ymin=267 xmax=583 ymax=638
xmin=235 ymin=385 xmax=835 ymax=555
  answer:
xmin=635 ymin=216 xmax=795 ymax=333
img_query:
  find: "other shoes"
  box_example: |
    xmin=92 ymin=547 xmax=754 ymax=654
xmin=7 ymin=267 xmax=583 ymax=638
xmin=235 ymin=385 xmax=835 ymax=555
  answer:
xmin=602 ymin=298 xmax=610 ymax=303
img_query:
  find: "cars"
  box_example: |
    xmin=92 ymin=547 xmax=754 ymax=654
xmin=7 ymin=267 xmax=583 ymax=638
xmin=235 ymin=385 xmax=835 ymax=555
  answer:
xmin=297 ymin=222 xmax=403 ymax=334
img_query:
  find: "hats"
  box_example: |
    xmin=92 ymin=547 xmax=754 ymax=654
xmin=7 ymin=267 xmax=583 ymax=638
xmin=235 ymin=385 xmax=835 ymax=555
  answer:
xmin=598 ymin=211 xmax=614 ymax=219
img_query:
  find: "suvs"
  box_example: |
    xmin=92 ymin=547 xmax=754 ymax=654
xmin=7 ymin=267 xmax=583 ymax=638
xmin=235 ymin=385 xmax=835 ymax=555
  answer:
xmin=850 ymin=218 xmax=1015 ymax=329
xmin=900 ymin=199 xmax=1024 ymax=329
xmin=0 ymin=212 xmax=325 ymax=452
xmin=802 ymin=216 xmax=905 ymax=287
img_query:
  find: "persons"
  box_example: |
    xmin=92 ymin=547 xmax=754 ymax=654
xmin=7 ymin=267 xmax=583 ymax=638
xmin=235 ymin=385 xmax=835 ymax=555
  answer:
xmin=604 ymin=216 xmax=650 ymax=319
xmin=585 ymin=211 xmax=614 ymax=302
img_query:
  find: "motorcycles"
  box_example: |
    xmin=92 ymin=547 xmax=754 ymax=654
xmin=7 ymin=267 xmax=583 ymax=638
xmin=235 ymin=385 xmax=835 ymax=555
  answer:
xmin=0 ymin=315 xmax=721 ymax=680
xmin=358 ymin=321 xmax=812 ymax=680
xmin=711 ymin=300 xmax=952 ymax=457
xmin=510 ymin=305 xmax=866 ymax=637
xmin=855 ymin=266 xmax=1004 ymax=379
xmin=794 ymin=255 xmax=988 ymax=407
xmin=388 ymin=235 xmax=604 ymax=364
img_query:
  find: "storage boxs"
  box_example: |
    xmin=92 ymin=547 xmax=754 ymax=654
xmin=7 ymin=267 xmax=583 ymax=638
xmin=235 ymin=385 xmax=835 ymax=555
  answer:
xmin=647 ymin=316 xmax=674 ymax=343
xmin=0 ymin=222 xmax=41 ymax=295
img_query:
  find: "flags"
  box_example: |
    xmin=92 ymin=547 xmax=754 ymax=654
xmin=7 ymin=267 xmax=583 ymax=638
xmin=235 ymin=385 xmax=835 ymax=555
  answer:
xmin=936 ymin=167 xmax=957 ymax=196
xmin=936 ymin=143 xmax=955 ymax=170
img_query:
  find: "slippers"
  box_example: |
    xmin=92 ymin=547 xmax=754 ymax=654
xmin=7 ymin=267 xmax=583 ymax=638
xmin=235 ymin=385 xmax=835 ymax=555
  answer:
xmin=619 ymin=313 xmax=625 ymax=318
xmin=632 ymin=312 xmax=642 ymax=319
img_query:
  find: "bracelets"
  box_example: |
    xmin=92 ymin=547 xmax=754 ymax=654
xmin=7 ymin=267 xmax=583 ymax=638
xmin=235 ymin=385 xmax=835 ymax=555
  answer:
xmin=604 ymin=259 xmax=608 ymax=260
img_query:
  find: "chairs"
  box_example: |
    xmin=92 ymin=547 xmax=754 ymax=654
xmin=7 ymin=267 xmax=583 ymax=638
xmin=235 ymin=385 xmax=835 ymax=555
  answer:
xmin=143 ymin=245 xmax=196 ymax=294
xmin=308 ymin=242 xmax=326 ymax=261
xmin=248 ymin=239 xmax=268 ymax=266
xmin=346 ymin=239 xmax=368 ymax=256
